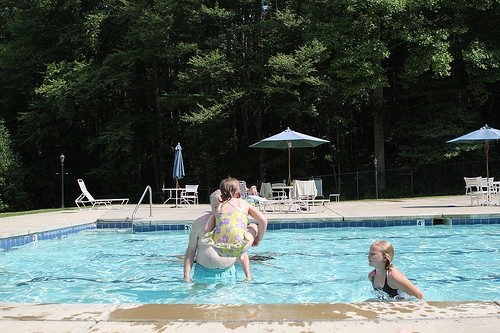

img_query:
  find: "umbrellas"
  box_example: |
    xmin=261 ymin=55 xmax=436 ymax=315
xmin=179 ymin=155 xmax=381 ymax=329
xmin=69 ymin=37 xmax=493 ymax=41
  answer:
xmin=250 ymin=127 xmax=330 ymax=185
xmin=170 ymin=142 xmax=185 ymax=208
xmin=446 ymin=124 xmax=500 ymax=206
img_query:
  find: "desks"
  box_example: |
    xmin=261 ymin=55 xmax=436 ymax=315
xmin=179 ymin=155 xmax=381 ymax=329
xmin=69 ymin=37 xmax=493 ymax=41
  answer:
xmin=272 ymin=186 xmax=293 ymax=199
xmin=487 ymin=182 xmax=500 ymax=206
xmin=162 ymin=188 xmax=191 ymax=205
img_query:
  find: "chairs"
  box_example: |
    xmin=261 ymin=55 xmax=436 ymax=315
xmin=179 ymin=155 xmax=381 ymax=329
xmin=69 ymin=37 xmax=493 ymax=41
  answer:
xmin=464 ymin=177 xmax=500 ymax=208
xmin=180 ymin=184 xmax=199 ymax=205
xmin=252 ymin=183 xmax=330 ymax=213
xmin=75 ymin=179 xmax=129 ymax=211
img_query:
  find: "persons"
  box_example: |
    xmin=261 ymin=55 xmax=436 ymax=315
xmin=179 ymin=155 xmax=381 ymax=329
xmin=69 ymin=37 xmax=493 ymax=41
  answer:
xmin=184 ymin=177 xmax=267 ymax=282
xmin=368 ymin=240 xmax=423 ymax=301
